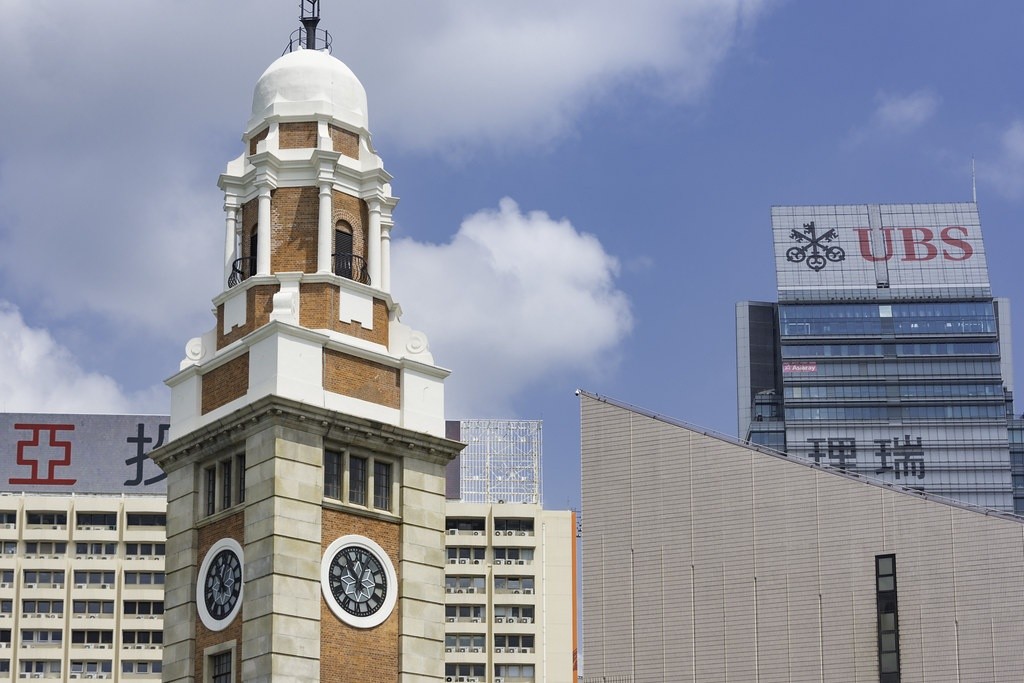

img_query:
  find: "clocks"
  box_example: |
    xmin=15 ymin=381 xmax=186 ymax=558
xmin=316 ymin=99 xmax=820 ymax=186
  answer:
xmin=321 ymin=534 xmax=397 ymax=629
xmin=196 ymin=537 xmax=246 ymax=632
